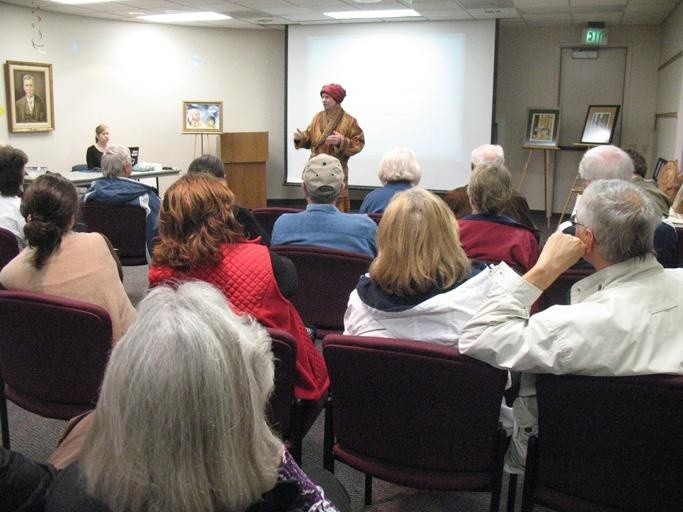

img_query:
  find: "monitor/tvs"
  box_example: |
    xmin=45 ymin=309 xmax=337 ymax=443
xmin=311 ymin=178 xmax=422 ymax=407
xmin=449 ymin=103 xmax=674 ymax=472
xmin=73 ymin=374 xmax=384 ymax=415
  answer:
xmin=127 ymin=146 xmax=139 ymax=166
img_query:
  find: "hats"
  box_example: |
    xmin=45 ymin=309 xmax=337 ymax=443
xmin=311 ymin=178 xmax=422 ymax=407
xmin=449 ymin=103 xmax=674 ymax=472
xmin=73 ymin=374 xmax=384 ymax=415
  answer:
xmin=320 ymin=82 xmax=347 ymax=104
xmin=301 ymin=153 xmax=345 ymax=196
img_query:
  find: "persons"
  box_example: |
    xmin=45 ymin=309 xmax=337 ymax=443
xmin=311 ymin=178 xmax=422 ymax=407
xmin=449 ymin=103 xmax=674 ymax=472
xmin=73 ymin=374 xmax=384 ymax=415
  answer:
xmin=0 ymin=171 xmax=138 ymax=347
xmin=293 ymin=84 xmax=365 ymax=214
xmin=272 ymin=153 xmax=379 ymax=258
xmin=207 ymin=115 xmax=215 ymax=129
xmin=149 ymin=173 xmax=330 ymax=441
xmin=189 ymin=110 xmax=205 ymax=129
xmin=42 ymin=279 xmax=339 ymax=512
xmin=16 ymin=74 xmax=47 ymax=123
xmin=342 ymin=187 xmax=517 ymax=449
xmin=0 ymin=408 xmax=95 ymax=512
xmin=0 ymin=146 xmax=29 ymax=253
xmin=187 ymin=154 xmax=271 ymax=246
xmin=358 ymin=147 xmax=421 ymax=214
xmin=86 ymin=124 xmax=109 ymax=170
xmin=438 ymin=144 xmax=683 ymax=476
xmin=79 ymin=144 xmax=161 ymax=266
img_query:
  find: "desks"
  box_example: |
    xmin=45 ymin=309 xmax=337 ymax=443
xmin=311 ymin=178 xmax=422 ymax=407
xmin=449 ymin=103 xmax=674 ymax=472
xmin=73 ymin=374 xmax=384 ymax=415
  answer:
xmin=24 ymin=162 xmax=181 ymax=196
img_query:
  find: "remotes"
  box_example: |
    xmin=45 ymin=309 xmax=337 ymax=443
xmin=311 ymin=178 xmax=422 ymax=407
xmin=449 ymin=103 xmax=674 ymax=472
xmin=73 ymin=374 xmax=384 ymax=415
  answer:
xmin=162 ymin=166 xmax=176 ymax=170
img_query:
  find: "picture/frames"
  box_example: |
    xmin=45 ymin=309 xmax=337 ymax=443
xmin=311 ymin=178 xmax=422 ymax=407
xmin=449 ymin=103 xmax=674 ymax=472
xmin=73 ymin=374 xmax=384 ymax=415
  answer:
xmin=523 ymin=108 xmax=562 ymax=150
xmin=581 ymin=105 xmax=622 ymax=147
xmin=5 ymin=59 xmax=55 ymax=134
xmin=181 ymin=99 xmax=224 ymax=135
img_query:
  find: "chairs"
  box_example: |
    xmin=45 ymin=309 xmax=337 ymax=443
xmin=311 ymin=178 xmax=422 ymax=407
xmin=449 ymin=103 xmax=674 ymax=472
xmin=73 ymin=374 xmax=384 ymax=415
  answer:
xmin=540 ymin=266 xmax=597 ymax=306
xmin=268 ymin=244 xmax=376 ymax=340
xmin=72 ymin=163 xmax=89 ymax=172
xmin=260 ymin=328 xmax=334 ymax=475
xmin=0 ymin=287 xmax=114 ymax=451
xmin=251 ymin=207 xmax=305 ymax=238
xmin=0 ymin=226 xmax=22 ymax=270
xmin=76 ymin=205 xmax=150 ymax=267
xmin=369 ymin=211 xmax=383 ymax=222
xmin=521 ymin=371 xmax=683 ymax=512
xmin=320 ymin=333 xmax=521 ymax=511
